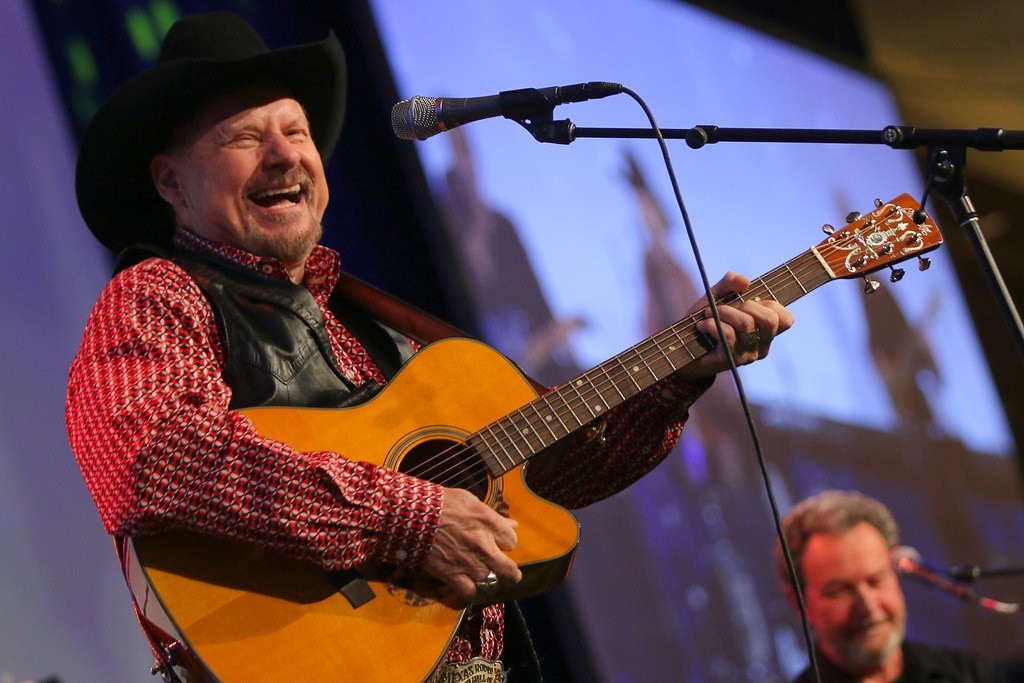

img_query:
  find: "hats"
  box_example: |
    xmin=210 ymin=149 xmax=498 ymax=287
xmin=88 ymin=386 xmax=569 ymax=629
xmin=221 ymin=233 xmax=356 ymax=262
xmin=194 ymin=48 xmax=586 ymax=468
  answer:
xmin=74 ymin=16 xmax=347 ymax=255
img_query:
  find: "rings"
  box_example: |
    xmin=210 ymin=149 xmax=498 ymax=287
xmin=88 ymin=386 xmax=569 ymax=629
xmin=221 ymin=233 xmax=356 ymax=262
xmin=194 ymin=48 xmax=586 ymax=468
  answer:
xmin=740 ymin=327 xmax=760 ymax=347
xmin=476 ymin=569 xmax=499 ymax=597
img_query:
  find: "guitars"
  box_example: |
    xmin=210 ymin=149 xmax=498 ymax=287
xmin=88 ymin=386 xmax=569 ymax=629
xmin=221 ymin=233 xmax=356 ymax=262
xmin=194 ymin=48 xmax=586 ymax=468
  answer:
xmin=117 ymin=188 xmax=947 ymax=683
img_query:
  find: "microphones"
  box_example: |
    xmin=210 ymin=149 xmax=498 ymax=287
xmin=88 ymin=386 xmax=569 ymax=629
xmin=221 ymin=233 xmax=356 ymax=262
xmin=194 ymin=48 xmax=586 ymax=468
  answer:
xmin=888 ymin=546 xmax=1014 ymax=613
xmin=391 ymin=80 xmax=623 ymax=141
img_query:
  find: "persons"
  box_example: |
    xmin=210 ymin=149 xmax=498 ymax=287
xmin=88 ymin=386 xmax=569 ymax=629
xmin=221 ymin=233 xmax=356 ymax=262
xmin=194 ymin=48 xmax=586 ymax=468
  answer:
xmin=839 ymin=203 xmax=943 ymax=435
xmin=628 ymin=157 xmax=736 ymax=405
xmin=64 ymin=11 xmax=793 ymax=683
xmin=434 ymin=124 xmax=580 ymax=390
xmin=775 ymin=489 xmax=1024 ymax=683
